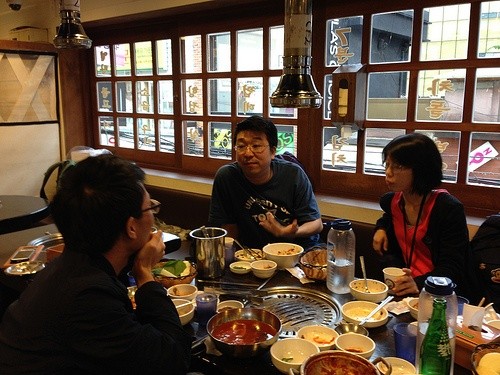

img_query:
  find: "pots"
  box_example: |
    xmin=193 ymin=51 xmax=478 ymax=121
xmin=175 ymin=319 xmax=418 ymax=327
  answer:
xmin=289 ymin=349 xmax=392 ymax=375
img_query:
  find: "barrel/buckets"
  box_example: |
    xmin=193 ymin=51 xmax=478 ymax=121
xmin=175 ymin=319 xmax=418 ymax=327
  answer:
xmin=187 ymin=227 xmax=228 ymax=279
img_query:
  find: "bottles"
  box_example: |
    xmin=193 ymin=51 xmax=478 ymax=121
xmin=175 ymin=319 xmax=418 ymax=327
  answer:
xmin=418 ymin=298 xmax=452 ymax=375
xmin=326 ymin=219 xmax=357 ymax=295
xmin=420 ymin=276 xmax=458 ymax=375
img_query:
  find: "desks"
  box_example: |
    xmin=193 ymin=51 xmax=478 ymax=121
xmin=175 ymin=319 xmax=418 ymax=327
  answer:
xmin=0 ymin=195 xmax=49 ymax=228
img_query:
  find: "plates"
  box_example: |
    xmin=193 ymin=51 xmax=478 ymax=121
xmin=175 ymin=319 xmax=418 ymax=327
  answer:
xmin=4 ymin=261 xmax=45 ymax=275
xmin=230 ymin=247 xmax=265 ymax=273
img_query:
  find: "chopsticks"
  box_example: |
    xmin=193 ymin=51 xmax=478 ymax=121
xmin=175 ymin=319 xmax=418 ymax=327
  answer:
xmin=229 ymin=237 xmax=262 ymax=261
xmin=358 ymin=295 xmax=394 ymax=326
xmin=175 ymin=300 xmax=195 ymax=308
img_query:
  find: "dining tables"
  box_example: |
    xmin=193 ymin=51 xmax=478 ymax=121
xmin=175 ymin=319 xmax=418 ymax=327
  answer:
xmin=0 ymin=223 xmax=358 ymax=306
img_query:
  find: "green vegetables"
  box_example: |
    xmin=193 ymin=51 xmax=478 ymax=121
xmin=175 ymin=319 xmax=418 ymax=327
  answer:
xmin=153 ymin=260 xmax=186 ymax=276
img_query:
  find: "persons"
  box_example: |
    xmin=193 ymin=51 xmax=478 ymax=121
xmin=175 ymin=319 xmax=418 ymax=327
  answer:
xmin=467 ymin=214 xmax=500 ymax=314
xmin=0 ymin=153 xmax=191 ymax=375
xmin=207 ymin=115 xmax=323 ymax=252
xmin=371 ymin=133 xmax=472 ymax=305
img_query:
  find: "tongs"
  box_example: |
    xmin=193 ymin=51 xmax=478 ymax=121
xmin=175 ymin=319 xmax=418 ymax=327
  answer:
xmin=198 ymin=281 xmax=269 ymax=301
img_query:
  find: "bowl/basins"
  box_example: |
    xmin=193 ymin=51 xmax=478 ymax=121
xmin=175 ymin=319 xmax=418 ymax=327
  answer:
xmin=471 ymin=341 xmax=500 ymax=375
xmin=251 ymin=242 xmax=304 ymax=276
xmin=167 ymin=282 xmax=282 ymax=358
xmin=409 ymin=297 xmax=432 ymax=318
xmin=270 ymin=323 xmax=377 ymax=372
xmin=378 ymin=356 xmax=416 ymax=375
xmin=342 ymin=300 xmax=390 ymax=327
xmin=349 ymin=278 xmax=390 ymax=302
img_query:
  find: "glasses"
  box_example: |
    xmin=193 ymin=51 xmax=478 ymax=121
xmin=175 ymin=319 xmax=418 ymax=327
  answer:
xmin=382 ymin=160 xmax=403 ymax=169
xmin=233 ymin=143 xmax=271 ymax=153
xmin=142 ymin=197 xmax=162 ymax=214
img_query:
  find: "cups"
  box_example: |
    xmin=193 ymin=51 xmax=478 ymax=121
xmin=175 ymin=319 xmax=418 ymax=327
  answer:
xmin=457 ymin=295 xmax=469 ymax=316
xmin=394 ymin=323 xmax=418 ymax=365
xmin=381 ymin=266 xmax=407 ymax=294
xmin=196 ymin=293 xmax=219 ymax=323
